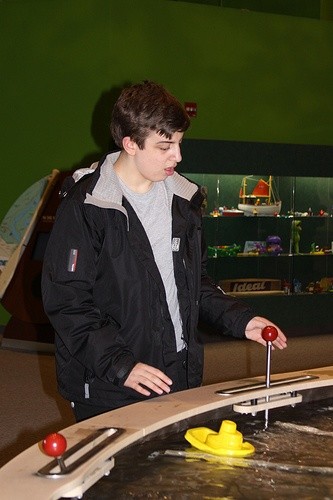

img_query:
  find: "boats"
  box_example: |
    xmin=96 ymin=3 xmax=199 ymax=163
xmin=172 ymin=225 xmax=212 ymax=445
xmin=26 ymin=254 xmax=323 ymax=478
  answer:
xmin=237 ymin=176 xmax=282 ymax=216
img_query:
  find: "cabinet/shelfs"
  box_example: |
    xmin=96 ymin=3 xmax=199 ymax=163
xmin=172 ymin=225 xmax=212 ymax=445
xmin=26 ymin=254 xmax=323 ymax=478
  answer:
xmin=174 ymin=138 xmax=333 ymax=341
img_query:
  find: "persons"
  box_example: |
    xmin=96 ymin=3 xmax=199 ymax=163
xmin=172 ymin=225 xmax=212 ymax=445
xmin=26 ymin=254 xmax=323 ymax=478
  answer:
xmin=41 ymin=80 xmax=287 ymax=423
